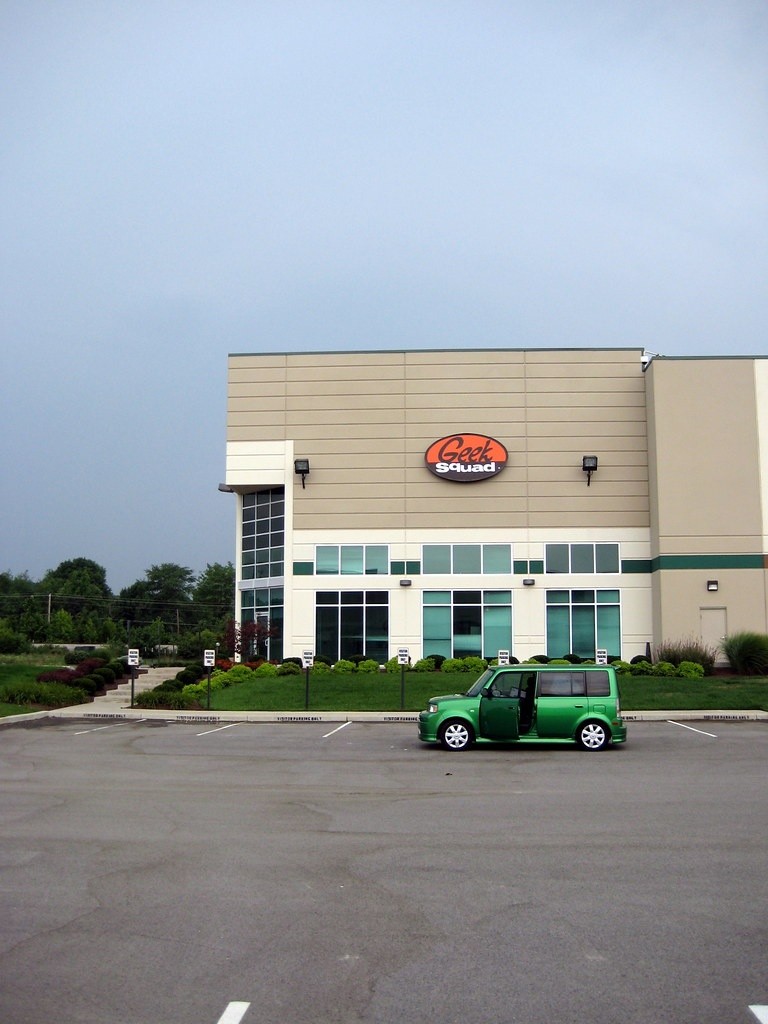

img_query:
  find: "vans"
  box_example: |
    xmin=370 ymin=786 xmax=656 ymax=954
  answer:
xmin=417 ymin=663 xmax=627 ymax=751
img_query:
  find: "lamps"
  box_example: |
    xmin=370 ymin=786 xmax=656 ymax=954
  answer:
xmin=295 ymin=458 xmax=311 ymax=489
xmin=400 ymin=580 xmax=411 ymax=586
xmin=217 ymin=483 xmax=234 ymax=493
xmin=583 ymin=455 xmax=598 ymax=486
xmin=707 ymin=581 xmax=719 ymax=591
xmin=523 ymin=579 xmax=535 ymax=584
